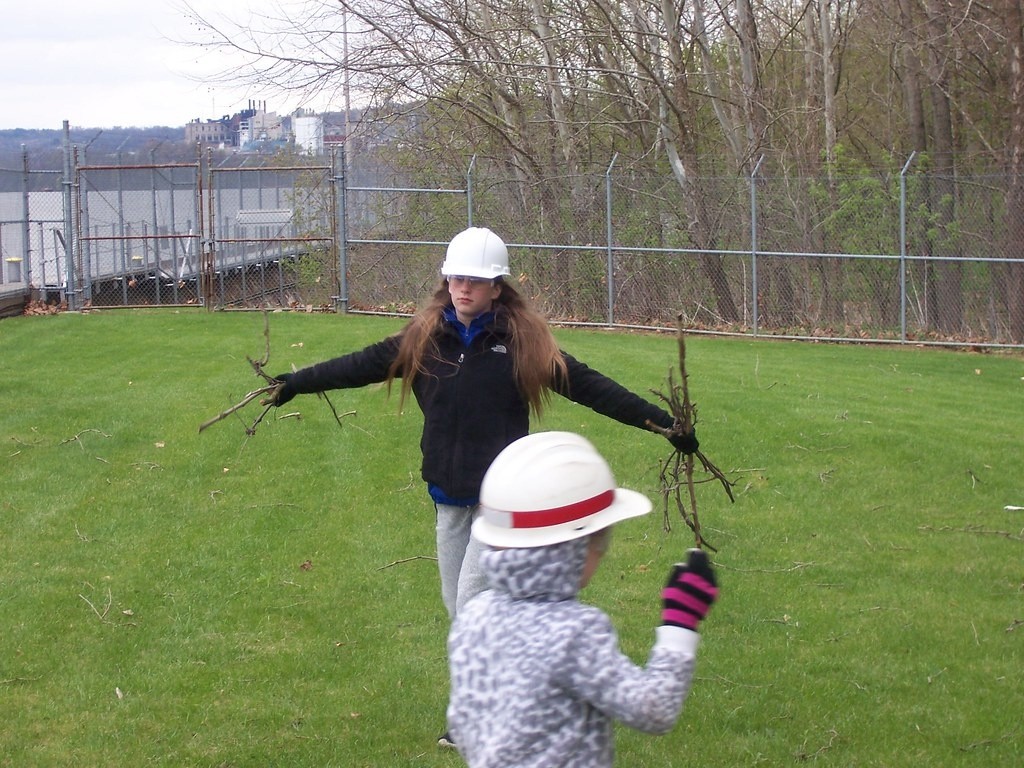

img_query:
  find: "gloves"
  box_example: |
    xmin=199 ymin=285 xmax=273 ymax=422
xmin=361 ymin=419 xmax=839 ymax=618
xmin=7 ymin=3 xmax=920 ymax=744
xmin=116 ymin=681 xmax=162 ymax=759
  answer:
xmin=654 ymin=411 xmax=699 ymax=455
xmin=660 ymin=549 xmax=718 ymax=632
xmin=269 ymin=373 xmax=298 ymax=407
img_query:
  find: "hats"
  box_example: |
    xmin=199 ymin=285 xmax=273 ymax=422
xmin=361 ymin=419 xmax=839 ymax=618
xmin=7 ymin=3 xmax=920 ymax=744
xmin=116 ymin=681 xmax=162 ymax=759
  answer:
xmin=471 ymin=431 xmax=652 ymax=548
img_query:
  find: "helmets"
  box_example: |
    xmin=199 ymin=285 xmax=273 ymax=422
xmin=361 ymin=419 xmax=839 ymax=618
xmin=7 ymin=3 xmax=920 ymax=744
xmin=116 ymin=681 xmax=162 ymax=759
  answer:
xmin=441 ymin=227 xmax=511 ymax=279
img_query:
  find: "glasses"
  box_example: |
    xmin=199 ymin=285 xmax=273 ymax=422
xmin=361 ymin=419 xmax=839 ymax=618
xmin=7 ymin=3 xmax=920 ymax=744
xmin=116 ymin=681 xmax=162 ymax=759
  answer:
xmin=447 ymin=275 xmax=494 ymax=289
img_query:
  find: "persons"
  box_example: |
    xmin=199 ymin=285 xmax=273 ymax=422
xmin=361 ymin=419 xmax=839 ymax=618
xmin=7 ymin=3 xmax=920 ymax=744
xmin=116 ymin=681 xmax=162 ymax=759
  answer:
xmin=446 ymin=429 xmax=721 ymax=768
xmin=266 ymin=227 xmax=700 ymax=744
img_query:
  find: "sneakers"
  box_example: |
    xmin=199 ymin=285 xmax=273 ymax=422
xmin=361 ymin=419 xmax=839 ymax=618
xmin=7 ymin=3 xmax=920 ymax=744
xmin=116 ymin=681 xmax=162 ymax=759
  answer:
xmin=438 ymin=733 xmax=457 ymax=747
